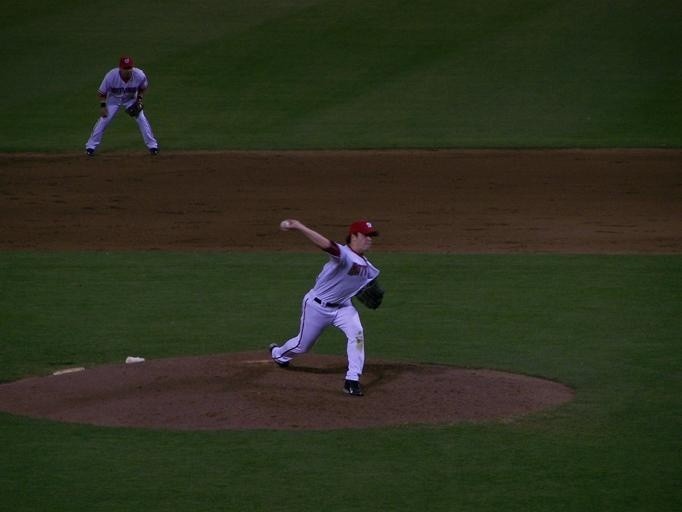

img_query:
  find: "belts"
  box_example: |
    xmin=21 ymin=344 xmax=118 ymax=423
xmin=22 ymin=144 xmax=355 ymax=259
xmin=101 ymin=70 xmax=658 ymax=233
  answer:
xmin=314 ymin=297 xmax=338 ymax=307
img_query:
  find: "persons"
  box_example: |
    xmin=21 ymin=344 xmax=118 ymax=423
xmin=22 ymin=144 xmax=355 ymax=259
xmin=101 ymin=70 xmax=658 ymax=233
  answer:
xmin=268 ymin=218 xmax=384 ymax=397
xmin=82 ymin=55 xmax=158 ymax=157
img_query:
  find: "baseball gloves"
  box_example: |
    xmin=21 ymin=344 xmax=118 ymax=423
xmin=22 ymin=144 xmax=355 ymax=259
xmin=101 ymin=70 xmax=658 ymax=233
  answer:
xmin=358 ymin=277 xmax=383 ymax=312
xmin=124 ymin=100 xmax=143 ymax=119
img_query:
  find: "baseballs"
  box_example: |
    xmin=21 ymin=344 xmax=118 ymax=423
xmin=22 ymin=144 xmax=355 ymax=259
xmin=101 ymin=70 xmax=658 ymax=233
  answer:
xmin=280 ymin=221 xmax=290 ymax=231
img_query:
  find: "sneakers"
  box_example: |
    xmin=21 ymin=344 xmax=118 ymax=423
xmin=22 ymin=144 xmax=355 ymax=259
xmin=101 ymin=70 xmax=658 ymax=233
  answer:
xmin=149 ymin=148 xmax=158 ymax=157
xmin=87 ymin=149 xmax=94 ymax=157
xmin=342 ymin=380 xmax=363 ymax=397
xmin=268 ymin=342 xmax=289 ymax=368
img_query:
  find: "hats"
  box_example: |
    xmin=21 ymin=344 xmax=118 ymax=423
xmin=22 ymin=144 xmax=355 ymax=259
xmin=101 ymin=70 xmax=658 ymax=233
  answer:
xmin=349 ymin=220 xmax=378 ymax=239
xmin=119 ymin=56 xmax=136 ymax=69
